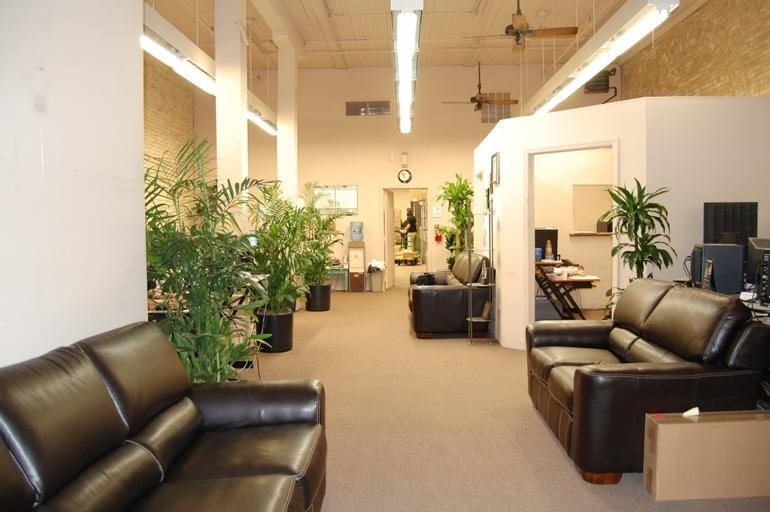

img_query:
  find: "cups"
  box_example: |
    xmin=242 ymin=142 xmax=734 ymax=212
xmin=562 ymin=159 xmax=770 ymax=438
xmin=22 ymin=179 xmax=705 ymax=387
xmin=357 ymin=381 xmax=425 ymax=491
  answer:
xmin=535 ymin=248 xmax=542 ymax=259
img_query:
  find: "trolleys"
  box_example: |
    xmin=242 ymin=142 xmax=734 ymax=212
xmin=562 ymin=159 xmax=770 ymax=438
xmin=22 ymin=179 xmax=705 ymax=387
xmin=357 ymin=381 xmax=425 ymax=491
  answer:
xmin=395 ymin=235 xmax=420 ymax=266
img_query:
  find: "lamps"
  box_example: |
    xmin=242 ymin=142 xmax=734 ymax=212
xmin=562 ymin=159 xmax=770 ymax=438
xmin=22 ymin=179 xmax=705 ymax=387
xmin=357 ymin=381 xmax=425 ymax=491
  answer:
xmin=142 ymin=0 xmax=278 ymax=137
xmin=390 ymin=0 xmax=425 ymax=135
xmin=521 ymin=1 xmax=680 ymax=117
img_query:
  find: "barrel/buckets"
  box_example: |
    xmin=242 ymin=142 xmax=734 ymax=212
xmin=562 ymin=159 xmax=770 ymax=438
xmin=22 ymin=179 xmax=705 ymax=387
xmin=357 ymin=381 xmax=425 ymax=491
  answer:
xmin=350 ymin=221 xmax=363 ymax=241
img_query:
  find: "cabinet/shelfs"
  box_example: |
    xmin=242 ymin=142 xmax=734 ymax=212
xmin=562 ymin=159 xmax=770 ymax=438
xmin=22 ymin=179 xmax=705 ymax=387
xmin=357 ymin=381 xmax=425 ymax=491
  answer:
xmin=410 ymin=199 xmax=426 ymax=264
xmin=466 ymin=282 xmax=497 ymax=346
xmin=310 ymin=184 xmax=358 ymax=215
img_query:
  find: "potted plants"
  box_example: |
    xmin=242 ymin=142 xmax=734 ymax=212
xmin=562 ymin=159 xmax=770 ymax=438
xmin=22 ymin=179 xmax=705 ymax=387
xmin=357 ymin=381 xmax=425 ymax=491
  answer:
xmin=244 ymin=138 xmax=354 ymax=353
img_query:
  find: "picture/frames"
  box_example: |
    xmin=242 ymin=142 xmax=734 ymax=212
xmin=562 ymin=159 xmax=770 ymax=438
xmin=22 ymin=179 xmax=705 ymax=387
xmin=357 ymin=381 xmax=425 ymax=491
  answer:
xmin=490 ymin=152 xmax=501 ymax=185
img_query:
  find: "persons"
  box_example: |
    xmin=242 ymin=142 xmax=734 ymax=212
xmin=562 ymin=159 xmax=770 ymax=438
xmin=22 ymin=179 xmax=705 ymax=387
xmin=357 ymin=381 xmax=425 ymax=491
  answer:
xmin=402 ymin=209 xmax=417 ymax=250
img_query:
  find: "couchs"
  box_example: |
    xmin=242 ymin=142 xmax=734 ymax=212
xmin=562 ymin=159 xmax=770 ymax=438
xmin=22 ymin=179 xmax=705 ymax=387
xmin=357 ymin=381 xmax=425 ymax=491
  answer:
xmin=525 ymin=279 xmax=770 ymax=485
xmin=1 ymin=322 xmax=328 ymax=512
xmin=407 ymin=251 xmax=496 ymax=339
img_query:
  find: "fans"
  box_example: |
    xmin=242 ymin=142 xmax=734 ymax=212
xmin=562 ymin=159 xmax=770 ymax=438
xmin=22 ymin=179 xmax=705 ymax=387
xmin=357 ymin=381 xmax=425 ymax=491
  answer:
xmin=461 ymin=0 xmax=577 ymax=54
xmin=442 ymin=61 xmax=518 ymax=112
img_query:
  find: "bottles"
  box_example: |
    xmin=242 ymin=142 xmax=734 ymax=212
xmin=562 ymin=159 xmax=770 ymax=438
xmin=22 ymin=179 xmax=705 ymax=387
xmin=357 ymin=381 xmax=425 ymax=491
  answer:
xmin=342 ymin=254 xmax=348 ymax=269
xmin=545 ymin=240 xmax=552 ymax=256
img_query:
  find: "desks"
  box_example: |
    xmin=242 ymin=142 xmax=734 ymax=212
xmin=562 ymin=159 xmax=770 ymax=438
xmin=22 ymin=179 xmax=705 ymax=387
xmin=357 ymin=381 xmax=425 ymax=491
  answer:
xmin=303 ymin=267 xmax=348 ymax=292
xmin=536 ymin=262 xmax=600 ymax=320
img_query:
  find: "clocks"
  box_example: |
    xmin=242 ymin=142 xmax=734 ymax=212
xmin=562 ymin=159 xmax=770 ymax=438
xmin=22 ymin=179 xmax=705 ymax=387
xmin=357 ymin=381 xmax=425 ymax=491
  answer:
xmin=398 ymin=169 xmax=412 ymax=184
